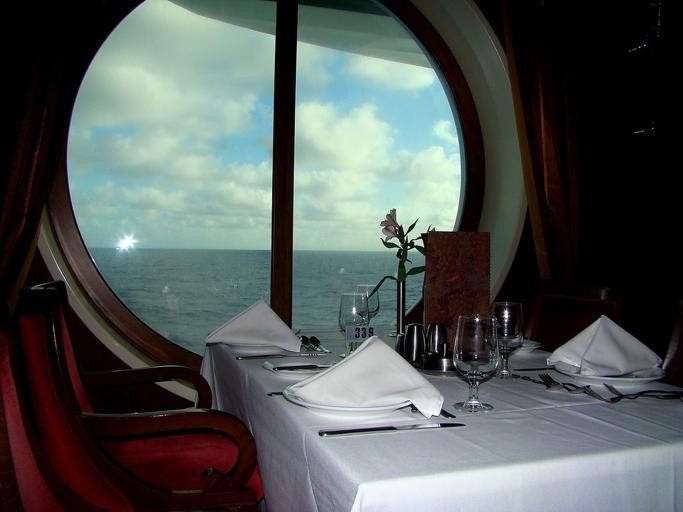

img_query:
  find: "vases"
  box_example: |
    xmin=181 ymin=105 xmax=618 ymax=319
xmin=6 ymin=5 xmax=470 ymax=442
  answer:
xmin=396 ymin=278 xmax=406 ymax=335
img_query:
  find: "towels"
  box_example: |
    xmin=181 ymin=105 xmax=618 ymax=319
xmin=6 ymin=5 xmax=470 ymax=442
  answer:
xmin=203 ymin=297 xmax=302 ymax=353
xmin=546 ymin=313 xmax=663 ymax=380
xmin=286 ymin=334 xmax=446 ymax=420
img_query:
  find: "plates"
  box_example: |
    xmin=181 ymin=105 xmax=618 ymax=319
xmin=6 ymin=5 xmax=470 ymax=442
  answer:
xmin=553 ymin=365 xmax=665 ymax=388
xmin=281 ymin=385 xmax=412 ymax=419
xmin=227 ymin=341 xmax=282 ymax=352
xmin=515 ymin=340 xmax=541 ymax=353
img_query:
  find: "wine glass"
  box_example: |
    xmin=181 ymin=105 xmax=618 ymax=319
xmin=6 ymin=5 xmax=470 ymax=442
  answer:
xmin=337 ymin=284 xmax=380 ymax=357
xmin=491 ymin=300 xmax=524 ymax=379
xmin=453 ymin=315 xmax=499 ymax=414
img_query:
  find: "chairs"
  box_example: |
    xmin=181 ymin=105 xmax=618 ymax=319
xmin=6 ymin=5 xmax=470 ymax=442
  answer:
xmin=524 ymin=278 xmax=627 ymax=351
xmin=657 ymin=296 xmax=682 ymax=389
xmin=0 ymin=319 xmax=125 ymax=510
xmin=17 ymin=280 xmax=265 ymax=511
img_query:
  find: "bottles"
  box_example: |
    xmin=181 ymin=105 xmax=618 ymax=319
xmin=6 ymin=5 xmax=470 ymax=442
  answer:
xmin=393 ymin=321 xmax=448 ymax=372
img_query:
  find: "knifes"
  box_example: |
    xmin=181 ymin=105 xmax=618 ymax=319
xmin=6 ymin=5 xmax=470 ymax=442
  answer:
xmin=235 ymin=351 xmax=327 ymax=360
xmin=318 ymin=421 xmax=466 ymax=438
xmin=272 ymin=365 xmax=332 ymax=371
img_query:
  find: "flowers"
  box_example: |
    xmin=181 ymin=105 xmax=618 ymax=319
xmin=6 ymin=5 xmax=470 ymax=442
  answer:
xmin=364 ymin=207 xmax=435 ymax=299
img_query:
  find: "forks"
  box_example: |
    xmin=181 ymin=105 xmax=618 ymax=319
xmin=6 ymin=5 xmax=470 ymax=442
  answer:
xmin=539 ymin=372 xmax=683 ymax=403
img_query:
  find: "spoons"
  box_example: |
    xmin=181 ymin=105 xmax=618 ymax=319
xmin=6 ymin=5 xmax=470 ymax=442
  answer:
xmin=296 ymin=334 xmax=332 ymax=353
xmin=511 ymin=374 xmax=563 ymax=391
